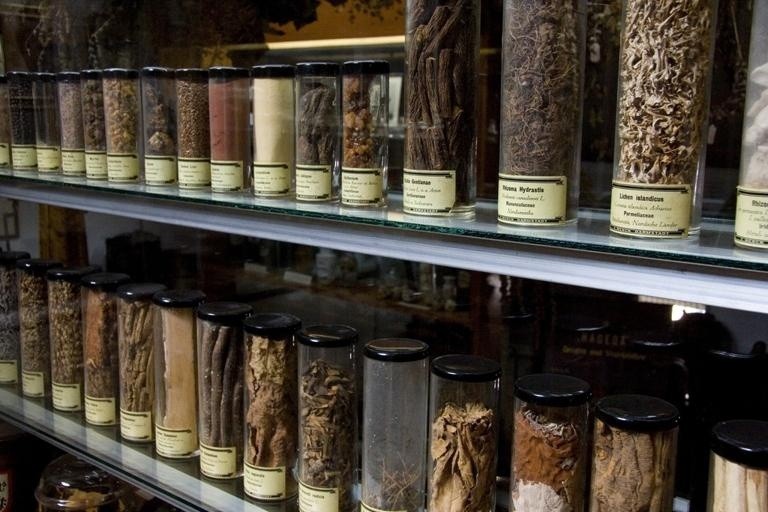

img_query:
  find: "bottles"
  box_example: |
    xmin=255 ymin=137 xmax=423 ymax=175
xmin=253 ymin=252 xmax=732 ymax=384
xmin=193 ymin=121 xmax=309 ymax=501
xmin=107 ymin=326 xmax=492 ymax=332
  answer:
xmin=0 ymin=248 xmax=767 ymax=511
xmin=0 ymin=1 xmax=768 ymax=249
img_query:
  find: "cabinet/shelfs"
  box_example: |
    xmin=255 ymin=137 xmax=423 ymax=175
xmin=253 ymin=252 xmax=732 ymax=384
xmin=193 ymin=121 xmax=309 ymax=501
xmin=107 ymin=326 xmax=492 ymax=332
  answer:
xmin=0 ymin=166 xmax=768 ymax=512
